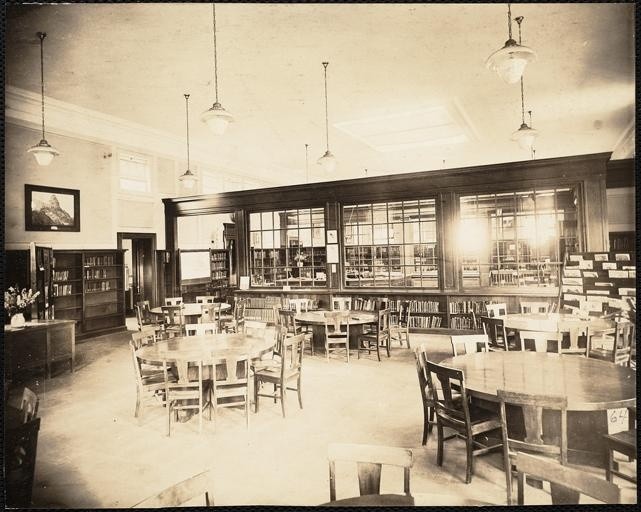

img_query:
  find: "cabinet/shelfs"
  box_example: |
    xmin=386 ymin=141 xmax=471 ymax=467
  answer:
xmin=83 ymin=248 xmax=128 ymax=340
xmin=561 ymin=249 xmax=637 ymax=331
xmin=50 ymin=249 xmax=84 ymax=342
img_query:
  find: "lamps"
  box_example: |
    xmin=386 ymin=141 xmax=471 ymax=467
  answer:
xmin=27 ymin=31 xmax=61 ymax=166
xmin=509 ymin=14 xmax=537 ymax=148
xmin=179 ymin=91 xmax=196 ymax=190
xmin=316 ymin=59 xmax=338 ymax=170
xmin=200 ymin=0 xmax=231 ymax=135
xmin=483 ymin=0 xmax=535 ymax=87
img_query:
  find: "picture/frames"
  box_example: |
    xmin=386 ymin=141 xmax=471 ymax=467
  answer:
xmin=24 ymin=182 xmax=81 ymax=233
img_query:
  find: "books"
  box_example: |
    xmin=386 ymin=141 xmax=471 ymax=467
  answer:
xmin=351 ymin=297 xmax=506 ymax=330
xmin=236 ymin=296 xmax=319 ymax=332
xmin=85 ymin=256 xmax=113 ymax=292
xmin=253 ymin=250 xmax=281 ymax=275
xmin=53 ymin=269 xmax=73 ymax=297
xmin=561 ymin=253 xmax=636 ymax=314
xmin=211 ymin=252 xmax=227 ymax=279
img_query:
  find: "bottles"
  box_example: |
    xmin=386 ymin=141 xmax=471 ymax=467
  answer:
xmin=84 ymin=267 xmax=107 ymax=280
xmin=52 ymin=268 xmax=70 ymax=281
xmin=85 ymin=255 xmax=113 ymax=266
xmin=87 ymin=280 xmax=110 ymax=292
xmin=53 ymin=257 xmax=57 ymax=269
xmin=53 ymin=283 xmax=73 ymax=296
